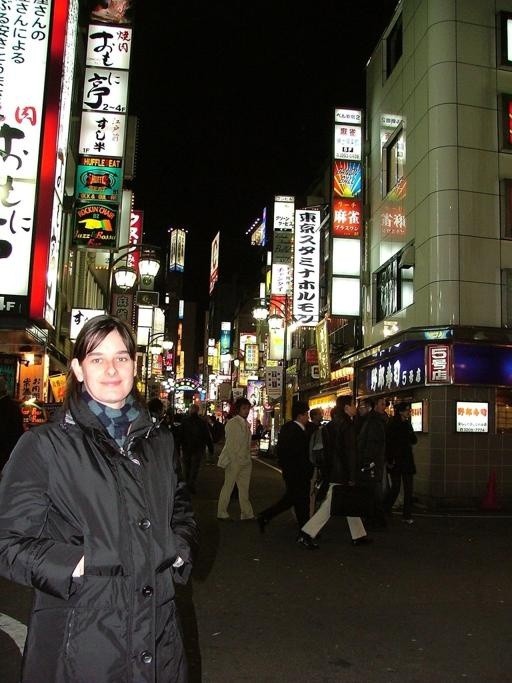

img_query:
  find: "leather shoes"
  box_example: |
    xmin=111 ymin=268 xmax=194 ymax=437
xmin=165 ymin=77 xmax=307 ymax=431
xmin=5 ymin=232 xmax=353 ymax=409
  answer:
xmin=295 ymin=536 xmax=319 ymax=549
xmin=259 ymin=515 xmax=269 ymax=533
xmin=352 ymin=539 xmax=373 ymax=546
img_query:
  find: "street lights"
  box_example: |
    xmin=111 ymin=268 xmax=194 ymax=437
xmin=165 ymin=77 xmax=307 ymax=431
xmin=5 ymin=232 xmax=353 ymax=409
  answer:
xmin=106 ymin=244 xmax=162 ymax=312
xmin=253 ymin=295 xmax=288 ymax=427
xmin=144 ymin=332 xmax=174 ymax=401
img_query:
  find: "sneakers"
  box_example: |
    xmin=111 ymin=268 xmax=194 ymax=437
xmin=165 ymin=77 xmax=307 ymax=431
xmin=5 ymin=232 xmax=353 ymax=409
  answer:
xmin=241 ymin=517 xmax=258 ymax=522
xmin=216 ymin=516 xmax=234 ymax=523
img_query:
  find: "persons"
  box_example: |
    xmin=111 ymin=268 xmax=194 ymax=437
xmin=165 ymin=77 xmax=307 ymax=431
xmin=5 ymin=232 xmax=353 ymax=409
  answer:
xmin=141 ymin=395 xmax=418 ymax=549
xmin=2 ymin=314 xmax=205 ymax=682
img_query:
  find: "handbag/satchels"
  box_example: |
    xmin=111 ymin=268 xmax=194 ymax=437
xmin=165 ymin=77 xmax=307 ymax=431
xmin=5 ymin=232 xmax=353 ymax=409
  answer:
xmin=331 ymin=485 xmax=368 ymax=516
